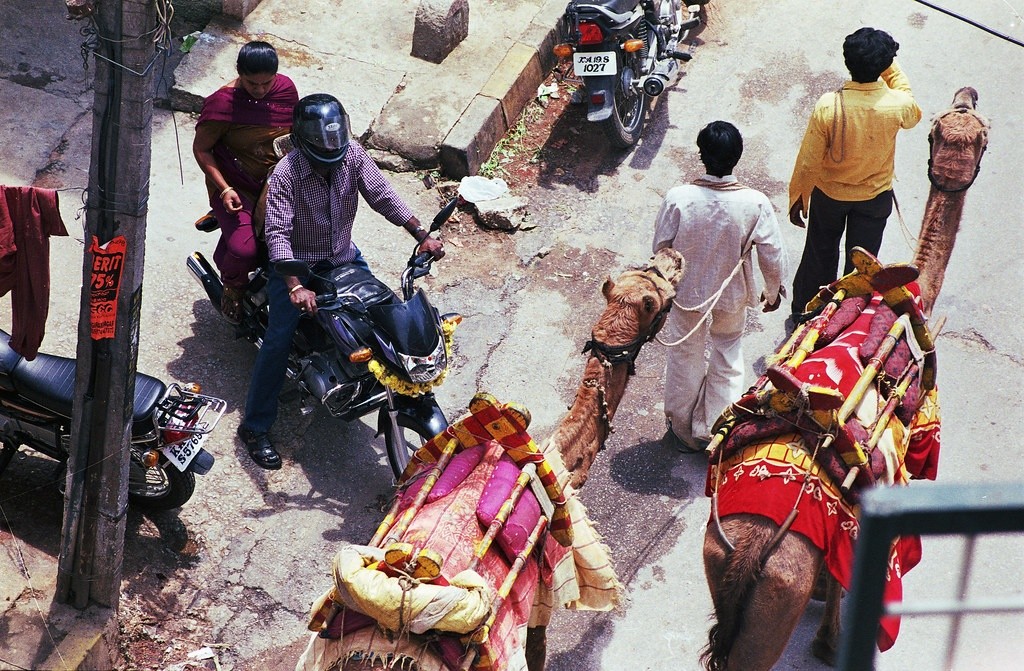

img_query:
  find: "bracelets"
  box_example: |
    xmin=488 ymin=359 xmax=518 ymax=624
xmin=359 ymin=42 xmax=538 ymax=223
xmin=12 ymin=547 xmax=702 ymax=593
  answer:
xmin=412 ymin=226 xmax=425 ymax=236
xmin=289 ymin=285 xmax=303 ymax=295
xmin=220 ymin=187 xmax=233 ymax=199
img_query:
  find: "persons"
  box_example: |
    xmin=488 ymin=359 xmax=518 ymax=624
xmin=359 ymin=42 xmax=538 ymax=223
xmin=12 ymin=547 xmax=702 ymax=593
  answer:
xmin=654 ymin=121 xmax=787 ymax=453
xmin=193 ymin=41 xmax=300 ymax=324
xmin=790 ymin=27 xmax=920 ymax=318
xmin=235 ymin=94 xmax=446 ymax=470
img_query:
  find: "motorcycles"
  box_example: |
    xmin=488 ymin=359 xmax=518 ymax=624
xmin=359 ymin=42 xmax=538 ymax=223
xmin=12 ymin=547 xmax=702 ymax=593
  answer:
xmin=1 ymin=327 xmax=228 ymax=510
xmin=550 ymin=1 xmax=702 ymax=149
xmin=187 ymin=197 xmax=449 ymax=485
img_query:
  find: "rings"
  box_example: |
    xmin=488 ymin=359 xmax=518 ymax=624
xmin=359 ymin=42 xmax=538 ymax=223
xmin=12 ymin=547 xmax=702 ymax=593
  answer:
xmin=441 ymin=248 xmax=444 ymax=251
xmin=301 ymin=307 xmax=305 ymax=311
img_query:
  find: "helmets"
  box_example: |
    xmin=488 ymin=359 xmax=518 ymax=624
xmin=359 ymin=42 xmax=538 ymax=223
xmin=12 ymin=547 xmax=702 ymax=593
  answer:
xmin=292 ymin=94 xmax=349 ymax=169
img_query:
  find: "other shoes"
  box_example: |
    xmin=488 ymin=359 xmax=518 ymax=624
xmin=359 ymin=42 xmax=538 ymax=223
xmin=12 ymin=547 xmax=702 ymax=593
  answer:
xmin=669 ymin=421 xmax=691 ymax=452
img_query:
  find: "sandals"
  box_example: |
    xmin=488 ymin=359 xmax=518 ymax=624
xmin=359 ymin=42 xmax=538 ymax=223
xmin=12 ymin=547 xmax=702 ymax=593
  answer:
xmin=237 ymin=424 xmax=281 ymax=469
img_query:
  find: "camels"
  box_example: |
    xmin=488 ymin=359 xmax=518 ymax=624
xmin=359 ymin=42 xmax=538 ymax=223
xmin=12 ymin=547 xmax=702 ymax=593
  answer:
xmin=318 ymin=248 xmax=685 ymax=671
xmin=699 ymin=88 xmax=989 ymax=671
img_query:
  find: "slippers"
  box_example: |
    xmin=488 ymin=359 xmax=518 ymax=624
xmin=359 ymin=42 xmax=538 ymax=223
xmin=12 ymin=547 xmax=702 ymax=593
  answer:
xmin=220 ymin=286 xmax=244 ymax=326
